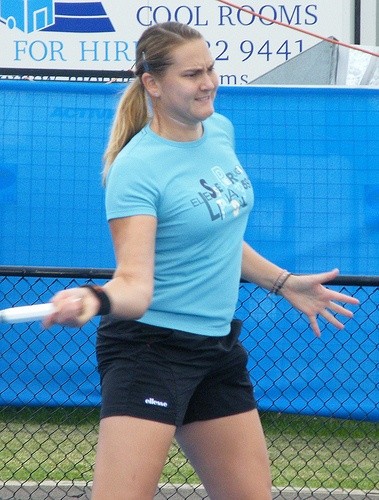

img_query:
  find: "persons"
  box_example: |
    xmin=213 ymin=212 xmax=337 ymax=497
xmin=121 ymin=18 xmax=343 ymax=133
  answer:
xmin=41 ymin=21 xmax=359 ymax=500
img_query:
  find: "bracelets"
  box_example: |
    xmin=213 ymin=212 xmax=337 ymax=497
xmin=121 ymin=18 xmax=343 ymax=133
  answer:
xmin=275 ymin=272 xmax=294 ymax=295
xmin=269 ymin=269 xmax=287 ymax=295
xmin=81 ymin=281 xmax=112 ymax=316
